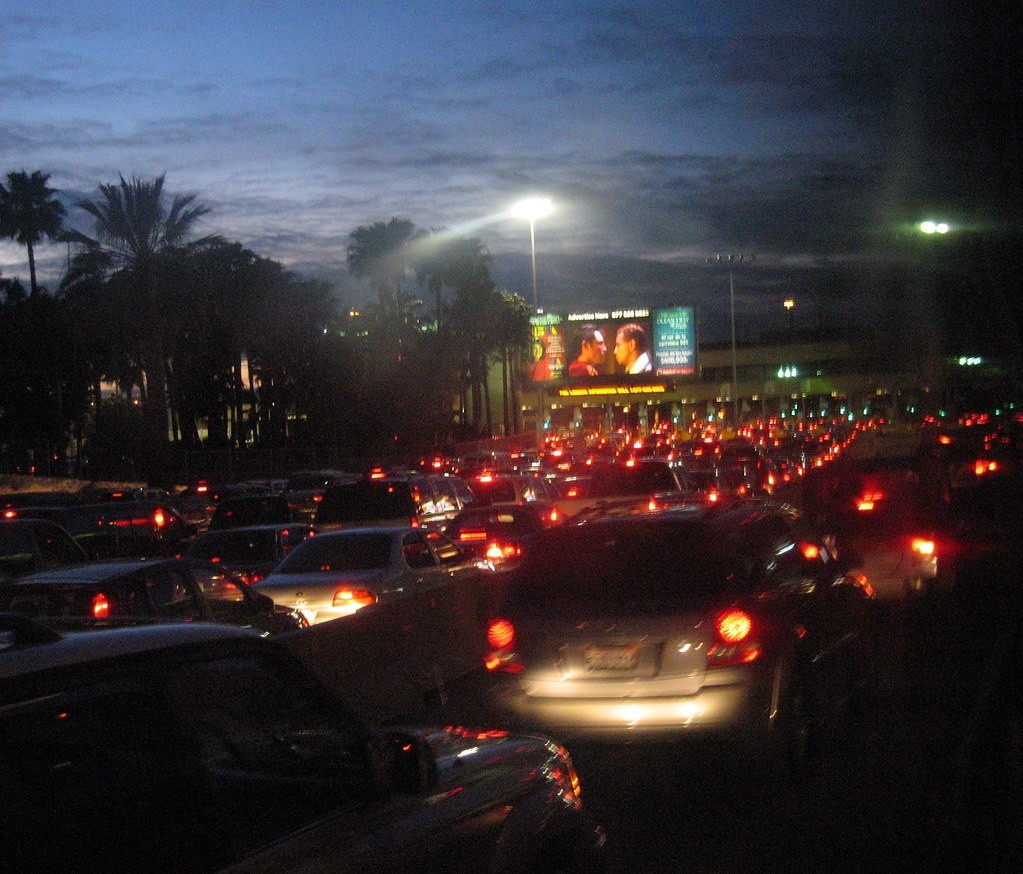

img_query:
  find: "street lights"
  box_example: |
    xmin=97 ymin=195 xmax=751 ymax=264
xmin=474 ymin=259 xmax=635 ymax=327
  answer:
xmin=512 ymin=189 xmax=550 ymax=318
xmin=705 ymin=252 xmax=747 ymax=420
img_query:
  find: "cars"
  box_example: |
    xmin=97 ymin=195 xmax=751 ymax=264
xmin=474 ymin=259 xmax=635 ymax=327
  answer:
xmin=1 ymin=412 xmax=1023 ymax=874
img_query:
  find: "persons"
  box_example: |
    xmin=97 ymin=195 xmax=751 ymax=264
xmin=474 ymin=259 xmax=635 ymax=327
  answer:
xmin=568 ymin=324 xmax=609 ymax=378
xmin=614 ymin=322 xmax=652 ymax=372
